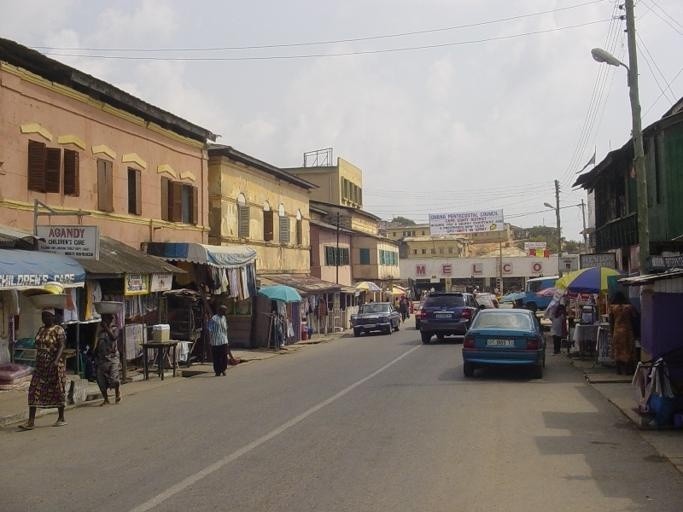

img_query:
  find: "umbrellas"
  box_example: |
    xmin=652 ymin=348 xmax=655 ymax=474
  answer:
xmin=353 ymin=281 xmax=382 ymax=291
xmin=258 ymin=286 xmax=302 ymax=303
xmin=555 ymin=266 xmax=624 ymax=295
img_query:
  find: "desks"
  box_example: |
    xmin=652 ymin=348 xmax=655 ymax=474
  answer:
xmin=141 ymin=341 xmax=178 ymax=381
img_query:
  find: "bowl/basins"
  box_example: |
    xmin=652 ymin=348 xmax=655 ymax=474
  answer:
xmin=92 ymin=301 xmax=123 ymax=314
xmin=29 ymin=294 xmax=67 ymax=309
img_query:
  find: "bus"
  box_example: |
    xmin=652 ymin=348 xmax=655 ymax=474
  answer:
xmin=522 ymin=276 xmax=560 ymax=311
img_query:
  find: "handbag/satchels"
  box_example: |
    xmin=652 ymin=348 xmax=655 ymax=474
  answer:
xmin=556 ymin=305 xmax=565 ymax=316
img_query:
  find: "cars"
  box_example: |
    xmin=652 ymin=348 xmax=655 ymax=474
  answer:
xmin=351 ymin=301 xmax=402 ymax=337
xmin=415 ymin=291 xmax=551 ymax=379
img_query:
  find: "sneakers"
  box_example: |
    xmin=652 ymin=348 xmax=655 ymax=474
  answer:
xmin=215 ymin=368 xmax=226 ymax=376
xmin=18 ymin=421 xmax=35 ymax=430
xmin=551 ymin=352 xmax=561 ymax=356
xmin=56 ymin=418 xmax=65 ymax=426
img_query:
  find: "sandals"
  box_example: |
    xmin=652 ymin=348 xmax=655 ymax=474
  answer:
xmin=100 ymin=391 xmax=121 ymax=406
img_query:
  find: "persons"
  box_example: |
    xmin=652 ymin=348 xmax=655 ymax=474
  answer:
xmin=18 ymin=307 xmax=68 ymax=429
xmin=609 ymin=292 xmax=639 ymax=375
xmin=93 ymin=314 xmax=121 ymax=406
xmin=208 ymin=305 xmax=229 ymax=376
xmin=542 ymin=294 xmax=567 ymax=355
xmin=395 ymin=295 xmax=409 ymax=324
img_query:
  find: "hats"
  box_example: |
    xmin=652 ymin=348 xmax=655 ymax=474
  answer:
xmin=42 ymin=305 xmax=55 ymax=317
xmin=220 ymin=304 xmax=227 ymax=309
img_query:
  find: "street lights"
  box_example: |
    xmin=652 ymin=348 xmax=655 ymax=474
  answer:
xmin=593 ymin=48 xmax=653 ymax=302
xmin=544 ymin=203 xmax=563 ymax=279
